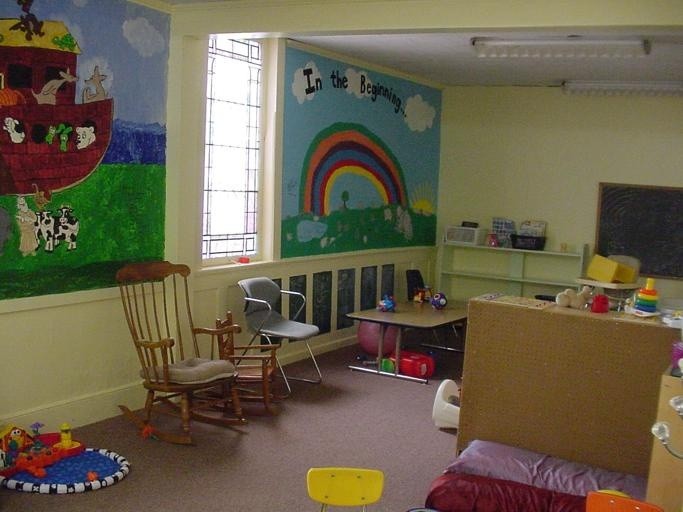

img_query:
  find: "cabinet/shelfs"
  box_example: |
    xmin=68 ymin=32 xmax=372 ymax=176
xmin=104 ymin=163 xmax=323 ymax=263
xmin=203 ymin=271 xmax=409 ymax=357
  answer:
xmin=434 ymin=232 xmax=589 ymax=313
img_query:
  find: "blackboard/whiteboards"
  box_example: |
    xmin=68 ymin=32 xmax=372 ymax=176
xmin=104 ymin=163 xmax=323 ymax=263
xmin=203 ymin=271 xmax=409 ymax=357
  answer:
xmin=595 ymin=182 xmax=682 ymax=281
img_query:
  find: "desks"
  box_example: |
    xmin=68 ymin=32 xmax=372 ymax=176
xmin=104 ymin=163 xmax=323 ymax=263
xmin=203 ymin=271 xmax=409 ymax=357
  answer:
xmin=345 ymin=299 xmax=468 ymax=383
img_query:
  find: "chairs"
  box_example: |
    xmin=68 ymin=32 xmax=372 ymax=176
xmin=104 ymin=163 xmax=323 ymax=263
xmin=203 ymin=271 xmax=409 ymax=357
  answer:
xmin=303 ymin=466 xmax=383 ymax=511
xmin=110 ymin=259 xmax=322 ymax=445
